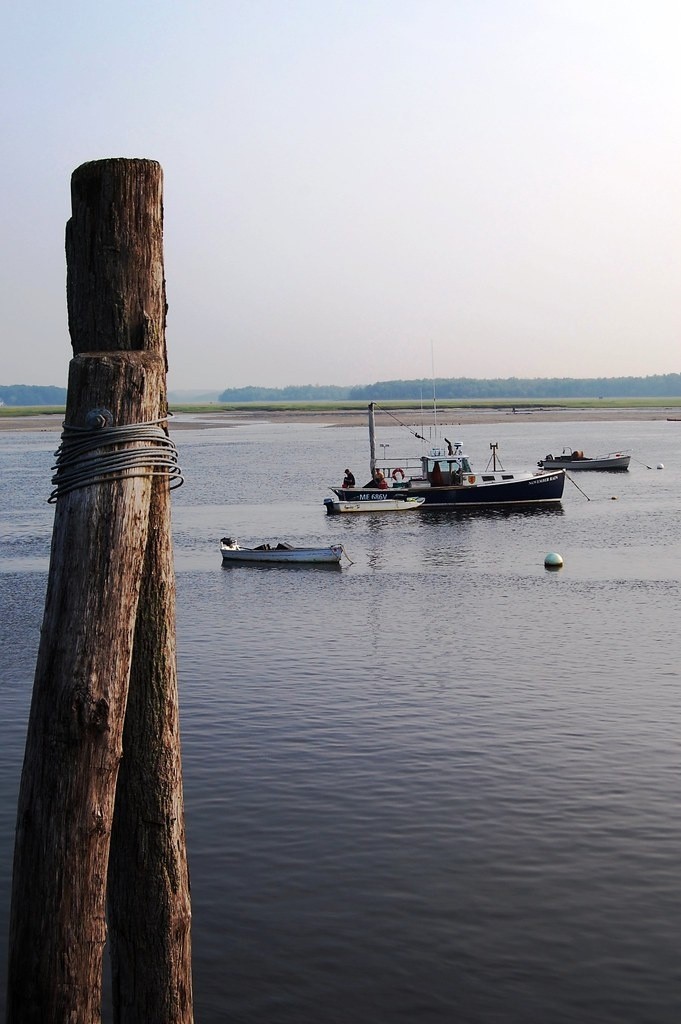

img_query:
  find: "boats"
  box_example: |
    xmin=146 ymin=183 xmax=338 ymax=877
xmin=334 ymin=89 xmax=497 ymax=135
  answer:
xmin=537 ymin=445 xmax=632 ymax=470
xmin=323 ymin=494 xmax=427 ymax=513
xmin=219 ymin=536 xmax=345 ymax=563
xmin=327 ymin=343 xmax=567 ymax=507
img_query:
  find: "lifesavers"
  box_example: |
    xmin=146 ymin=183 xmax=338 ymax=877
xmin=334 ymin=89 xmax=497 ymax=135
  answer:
xmin=392 ymin=467 xmax=405 ymax=482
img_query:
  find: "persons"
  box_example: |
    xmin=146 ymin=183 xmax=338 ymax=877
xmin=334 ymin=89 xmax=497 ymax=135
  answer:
xmin=375 ymin=468 xmax=384 ymax=480
xmin=343 ymin=469 xmax=355 ymax=488
